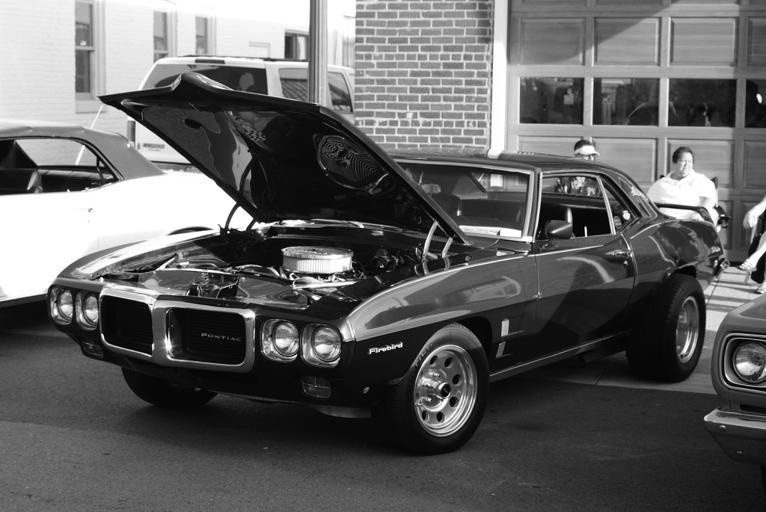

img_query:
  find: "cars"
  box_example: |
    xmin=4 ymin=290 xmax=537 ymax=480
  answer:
xmin=704 ymin=291 xmax=766 ymax=468
xmin=517 ymin=73 xmax=766 ymax=128
xmin=1 ymin=126 xmax=273 ymax=307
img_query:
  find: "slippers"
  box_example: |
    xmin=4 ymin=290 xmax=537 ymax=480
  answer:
xmin=738 ymin=262 xmax=757 ymax=271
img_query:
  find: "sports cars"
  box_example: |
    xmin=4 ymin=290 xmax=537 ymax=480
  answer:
xmin=46 ymin=66 xmax=724 ymax=456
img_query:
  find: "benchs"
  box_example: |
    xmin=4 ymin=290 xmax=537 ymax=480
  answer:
xmin=464 ymin=199 xmax=612 ymax=238
xmin=37 ymin=169 xmax=113 ymax=192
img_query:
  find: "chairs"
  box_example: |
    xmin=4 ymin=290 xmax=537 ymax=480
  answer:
xmin=656 ymin=173 xmax=733 ymax=273
xmin=538 ymin=202 xmax=578 ymax=238
xmin=429 ymin=192 xmax=464 ymax=216
xmin=0 ymin=168 xmax=44 ymax=194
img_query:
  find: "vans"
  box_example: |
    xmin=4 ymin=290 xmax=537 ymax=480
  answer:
xmin=124 ymin=56 xmax=357 ymax=173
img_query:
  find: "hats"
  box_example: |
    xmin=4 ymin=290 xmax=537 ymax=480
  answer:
xmin=574 ymin=145 xmax=600 ymax=157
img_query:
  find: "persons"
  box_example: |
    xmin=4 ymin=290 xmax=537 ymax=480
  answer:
xmin=554 ymin=139 xmax=603 ymax=197
xmin=737 ymin=194 xmax=766 ymax=294
xmin=645 ymin=146 xmax=722 ymax=233
